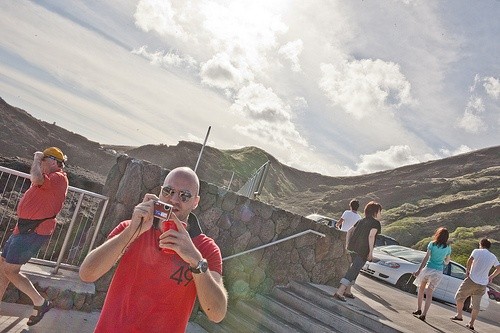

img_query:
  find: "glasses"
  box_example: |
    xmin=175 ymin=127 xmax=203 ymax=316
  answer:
xmin=161 ymin=186 xmax=196 ymax=203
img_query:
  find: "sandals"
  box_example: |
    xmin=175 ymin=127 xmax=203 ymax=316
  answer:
xmin=27 ymin=298 xmax=53 ymax=326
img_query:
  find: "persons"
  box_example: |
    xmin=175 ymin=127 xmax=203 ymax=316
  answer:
xmin=333 ymin=200 xmax=382 ymax=301
xmin=450 ymin=238 xmax=500 ymax=329
xmin=411 ymin=226 xmax=452 ymax=321
xmin=335 ymin=198 xmax=363 ymax=232
xmin=79 ymin=166 xmax=228 ymax=332
xmin=0 ymin=145 xmax=68 ymax=327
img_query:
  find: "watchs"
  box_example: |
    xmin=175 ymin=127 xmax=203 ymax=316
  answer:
xmin=190 ymin=258 xmax=210 ymax=274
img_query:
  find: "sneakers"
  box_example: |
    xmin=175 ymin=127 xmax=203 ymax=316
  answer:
xmin=343 ymin=291 xmax=355 ymax=299
xmin=334 ymin=293 xmax=346 ymax=302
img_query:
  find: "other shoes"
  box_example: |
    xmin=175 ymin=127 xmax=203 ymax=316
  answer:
xmin=412 ymin=309 xmax=425 ymax=321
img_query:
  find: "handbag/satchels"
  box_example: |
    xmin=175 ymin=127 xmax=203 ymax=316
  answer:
xmin=18 ymin=215 xmax=56 ymax=234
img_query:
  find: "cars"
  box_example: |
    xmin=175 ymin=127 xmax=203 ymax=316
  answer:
xmin=487 ymin=282 xmax=500 ymax=303
xmin=350 ymin=244 xmax=491 ymax=313
xmin=306 ymin=214 xmax=342 ymax=230
xmin=371 ymin=233 xmax=400 ymax=247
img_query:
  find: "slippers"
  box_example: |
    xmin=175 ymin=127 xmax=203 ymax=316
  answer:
xmin=450 ymin=315 xmax=463 ymax=321
xmin=466 ymin=323 xmax=474 ymax=330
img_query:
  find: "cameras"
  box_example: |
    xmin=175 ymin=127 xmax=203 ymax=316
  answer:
xmin=152 ymin=200 xmax=173 ymax=221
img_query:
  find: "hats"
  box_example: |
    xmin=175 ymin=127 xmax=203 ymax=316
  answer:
xmin=43 ymin=147 xmax=64 ymax=163
